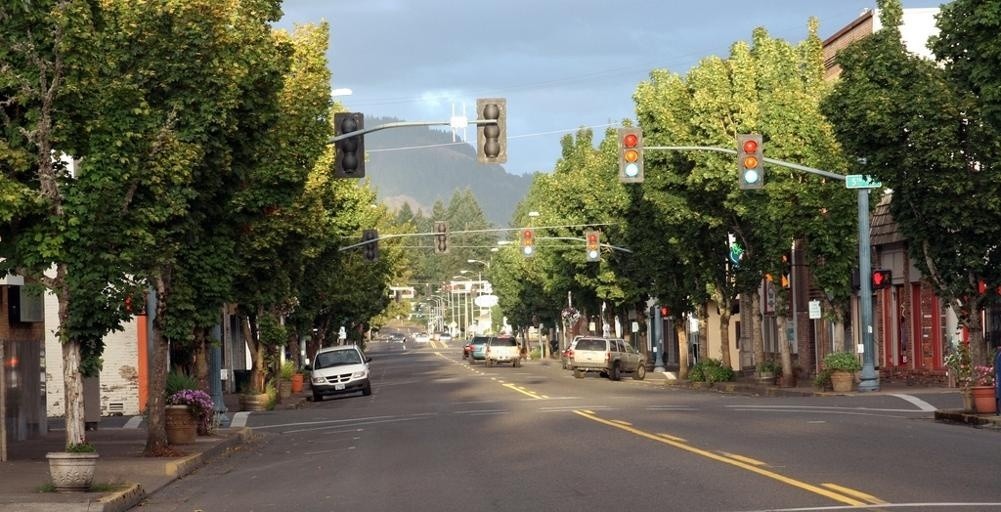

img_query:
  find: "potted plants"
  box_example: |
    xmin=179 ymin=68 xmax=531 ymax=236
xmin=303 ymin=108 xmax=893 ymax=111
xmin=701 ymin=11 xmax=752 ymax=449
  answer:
xmin=813 ymin=350 xmax=861 ymax=392
xmin=239 ymin=361 xmax=312 ymax=412
xmin=754 ymin=360 xmax=783 ymax=385
xmin=44 ymin=437 xmax=100 ymax=493
xmin=700 ymin=356 xmax=722 ymax=381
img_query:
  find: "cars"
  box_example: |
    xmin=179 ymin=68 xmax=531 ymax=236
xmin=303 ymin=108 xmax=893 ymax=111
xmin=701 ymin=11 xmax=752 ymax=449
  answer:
xmin=462 ymin=333 xmax=521 ymax=369
xmin=302 ymin=345 xmax=373 ymax=403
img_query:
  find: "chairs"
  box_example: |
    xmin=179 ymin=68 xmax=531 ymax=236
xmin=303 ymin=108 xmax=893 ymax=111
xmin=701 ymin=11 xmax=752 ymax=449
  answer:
xmin=320 ymin=353 xmax=354 ymax=365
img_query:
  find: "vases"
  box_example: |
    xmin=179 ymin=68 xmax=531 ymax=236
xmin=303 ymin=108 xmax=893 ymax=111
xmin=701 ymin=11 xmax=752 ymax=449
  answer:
xmin=958 ymin=385 xmax=997 ymax=414
xmin=165 ymin=404 xmax=201 ymax=446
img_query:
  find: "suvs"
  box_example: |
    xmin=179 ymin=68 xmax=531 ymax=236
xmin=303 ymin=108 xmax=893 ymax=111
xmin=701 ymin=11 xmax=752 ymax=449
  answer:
xmin=569 ymin=335 xmax=645 ymax=381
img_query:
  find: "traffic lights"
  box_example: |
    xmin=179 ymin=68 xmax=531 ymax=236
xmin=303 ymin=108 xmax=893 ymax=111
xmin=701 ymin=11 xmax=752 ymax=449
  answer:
xmin=517 ymin=227 xmax=536 ymax=260
xmin=584 ymin=228 xmax=600 ymax=263
xmin=735 ymin=128 xmax=765 ymax=195
xmin=615 ymin=125 xmax=647 ymax=189
xmin=659 ymin=304 xmax=673 ymax=318
xmin=872 ymin=267 xmax=891 ymax=289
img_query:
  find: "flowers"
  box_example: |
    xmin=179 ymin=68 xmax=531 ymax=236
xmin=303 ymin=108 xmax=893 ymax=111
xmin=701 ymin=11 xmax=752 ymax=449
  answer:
xmin=167 ymin=367 xmax=216 ymax=437
xmin=955 ymin=362 xmax=996 ymax=392
